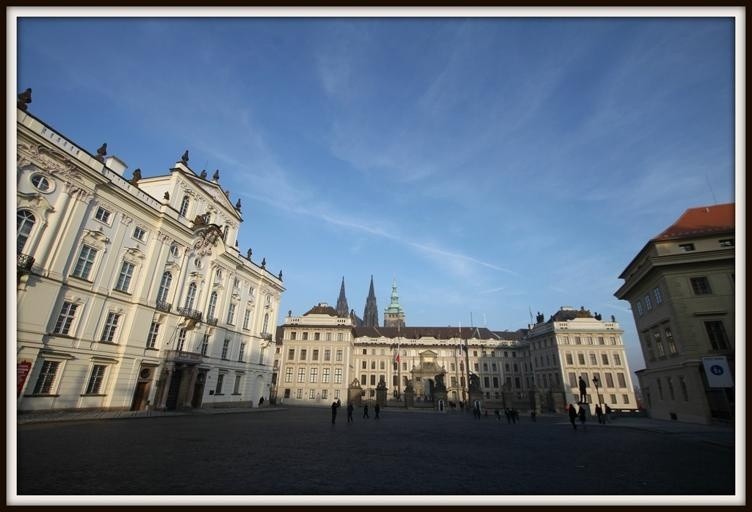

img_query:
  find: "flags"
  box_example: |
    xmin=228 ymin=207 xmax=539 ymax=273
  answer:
xmin=395 ymin=353 xmax=400 ymax=364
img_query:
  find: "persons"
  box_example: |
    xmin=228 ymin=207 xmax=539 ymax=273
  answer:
xmin=530 ymin=410 xmax=535 ymax=422
xmin=258 ymin=396 xmax=264 ymax=408
xmin=578 ymin=376 xmax=586 ymax=404
xmin=568 ymin=403 xmax=577 ymax=429
xmin=495 ymin=408 xmax=500 ymax=423
xmin=515 ymin=407 xmax=520 ymax=422
xmin=363 ymin=402 xmax=369 ymax=419
xmin=476 ymin=407 xmax=481 ymax=419
xmin=605 ymin=403 xmax=611 ymax=423
xmin=375 ymin=403 xmax=381 ymax=420
xmin=331 ymin=400 xmax=340 ymax=424
xmin=510 ymin=407 xmax=516 ymax=423
xmin=499 ymin=407 xmax=506 ymax=424
xmin=347 ymin=401 xmax=353 ymax=423
xmin=505 ymin=407 xmax=511 ymax=423
xmin=595 ymin=404 xmax=602 ymax=424
xmin=473 ymin=407 xmax=476 ymax=418
xmin=577 ymin=405 xmax=585 ymax=424
